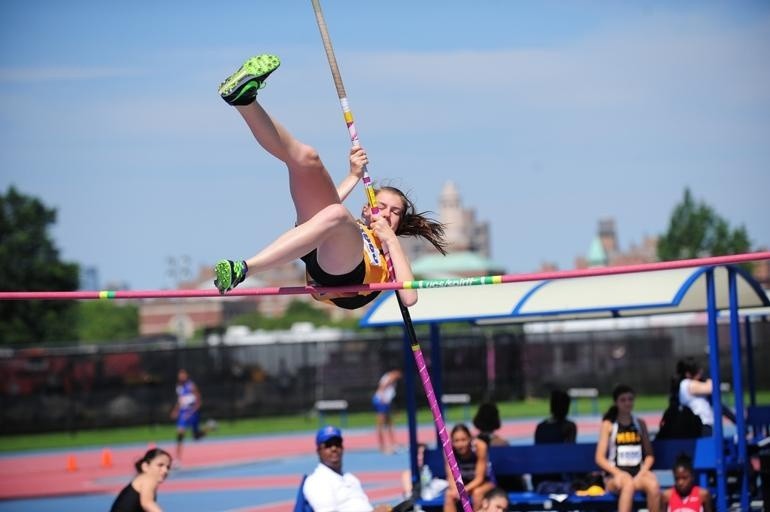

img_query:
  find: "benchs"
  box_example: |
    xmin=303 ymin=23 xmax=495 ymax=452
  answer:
xmin=414 ymin=437 xmax=716 ymax=506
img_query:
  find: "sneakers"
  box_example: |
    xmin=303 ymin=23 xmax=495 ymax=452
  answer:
xmin=212 ymin=257 xmax=250 ymax=295
xmin=217 ymin=53 xmax=282 ymax=107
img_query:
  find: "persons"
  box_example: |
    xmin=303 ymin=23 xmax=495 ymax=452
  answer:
xmin=169 ymin=365 xmax=218 ymax=469
xmin=213 ymin=53 xmax=451 ymax=310
xmin=110 ymin=448 xmax=172 ymax=512
xmin=292 ymin=355 xmax=766 ymax=511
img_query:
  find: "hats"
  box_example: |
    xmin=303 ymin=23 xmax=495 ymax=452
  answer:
xmin=313 ymin=424 xmax=344 ymax=447
